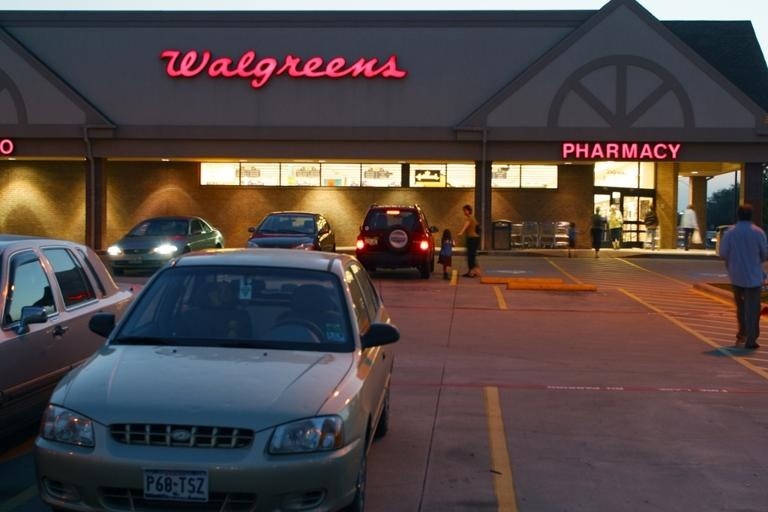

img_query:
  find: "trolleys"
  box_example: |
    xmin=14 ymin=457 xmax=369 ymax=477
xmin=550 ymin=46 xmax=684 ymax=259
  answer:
xmin=509 ymin=221 xmax=572 ymax=248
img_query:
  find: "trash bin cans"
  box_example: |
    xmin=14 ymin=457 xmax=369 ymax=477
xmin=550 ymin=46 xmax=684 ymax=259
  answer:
xmin=677 ymin=228 xmax=694 ymax=251
xmin=705 ymin=231 xmax=717 ymax=249
xmin=492 ymin=220 xmax=512 ymax=250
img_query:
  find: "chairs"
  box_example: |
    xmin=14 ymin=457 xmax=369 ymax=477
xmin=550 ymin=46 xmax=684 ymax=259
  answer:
xmin=510 ymin=217 xmax=570 ymax=250
xmin=183 ymin=279 xmax=253 ymax=342
xmin=272 ymin=283 xmax=343 ymax=343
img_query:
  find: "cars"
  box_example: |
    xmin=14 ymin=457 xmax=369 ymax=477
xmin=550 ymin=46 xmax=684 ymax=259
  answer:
xmin=0 ymin=233 xmax=144 ymax=448
xmin=32 ymin=245 xmax=400 ymax=512
xmin=244 ymin=210 xmax=337 ymax=255
xmin=703 ymin=230 xmax=716 ymax=248
xmin=107 ymin=215 xmax=225 ymax=278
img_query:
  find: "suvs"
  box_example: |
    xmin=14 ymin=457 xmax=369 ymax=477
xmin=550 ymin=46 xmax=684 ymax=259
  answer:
xmin=356 ymin=201 xmax=440 ymax=280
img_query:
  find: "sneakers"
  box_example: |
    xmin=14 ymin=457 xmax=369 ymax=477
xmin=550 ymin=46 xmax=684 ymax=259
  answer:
xmin=735 ymin=332 xmax=761 ymax=350
xmin=442 ymin=269 xmax=482 ymax=279
xmin=567 ymin=242 xmax=656 ymax=259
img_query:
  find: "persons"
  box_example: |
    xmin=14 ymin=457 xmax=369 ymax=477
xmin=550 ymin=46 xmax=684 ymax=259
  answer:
xmin=457 ymin=204 xmax=481 ymax=277
xmin=720 ymin=203 xmax=768 ymax=349
xmin=437 ymin=229 xmax=455 ymax=280
xmin=642 ymin=203 xmax=659 ymax=251
xmin=582 ymin=207 xmax=608 ymax=258
xmin=565 ymin=221 xmax=581 ymax=257
xmin=605 ymin=203 xmax=623 ymax=249
xmin=680 ymin=204 xmax=697 ymax=249
